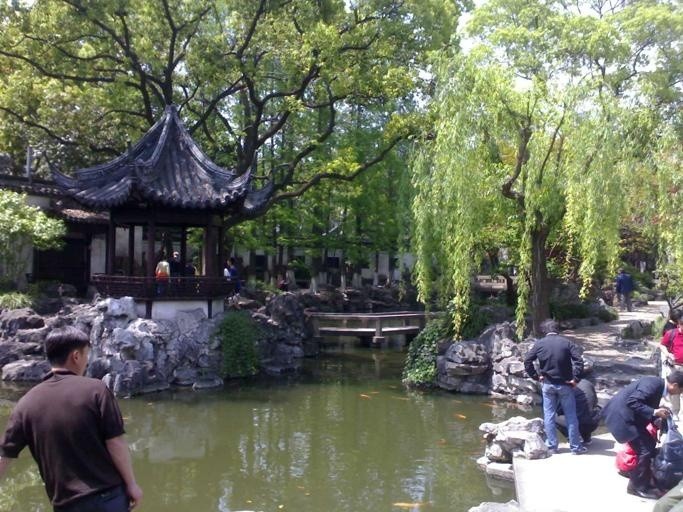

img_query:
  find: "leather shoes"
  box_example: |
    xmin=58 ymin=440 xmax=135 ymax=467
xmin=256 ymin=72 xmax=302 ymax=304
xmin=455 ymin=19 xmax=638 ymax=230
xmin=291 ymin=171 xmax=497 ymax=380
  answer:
xmin=627 ymin=486 xmax=656 ymax=498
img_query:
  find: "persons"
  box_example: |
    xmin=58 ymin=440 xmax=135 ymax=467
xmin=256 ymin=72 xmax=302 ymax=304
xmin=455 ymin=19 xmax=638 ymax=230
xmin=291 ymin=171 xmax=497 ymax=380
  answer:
xmin=552 ymin=376 xmax=601 ymax=446
xmin=599 ymin=369 xmax=683 ymax=499
xmin=226 ymin=256 xmax=241 ymax=313
xmin=153 ymin=250 xmax=196 ymax=298
xmin=661 ymin=307 xmax=683 ymax=336
xmin=0 ymin=324 xmax=142 ymax=511
xmin=614 ymin=268 xmax=637 ymax=312
xmin=522 ymin=317 xmax=588 ymax=456
xmin=658 ymin=308 xmax=682 ymax=421
xmin=615 ymin=417 xmax=668 ymax=477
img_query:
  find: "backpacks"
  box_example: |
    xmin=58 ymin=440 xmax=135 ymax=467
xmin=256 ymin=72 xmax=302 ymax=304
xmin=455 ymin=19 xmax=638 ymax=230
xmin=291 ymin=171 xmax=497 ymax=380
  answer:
xmin=652 ymin=417 xmax=683 ymax=488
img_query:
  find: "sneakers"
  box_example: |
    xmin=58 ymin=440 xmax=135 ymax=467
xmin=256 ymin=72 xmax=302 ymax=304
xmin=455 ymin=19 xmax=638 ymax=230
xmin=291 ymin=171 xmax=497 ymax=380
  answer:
xmin=548 ymin=435 xmax=592 ymax=455
